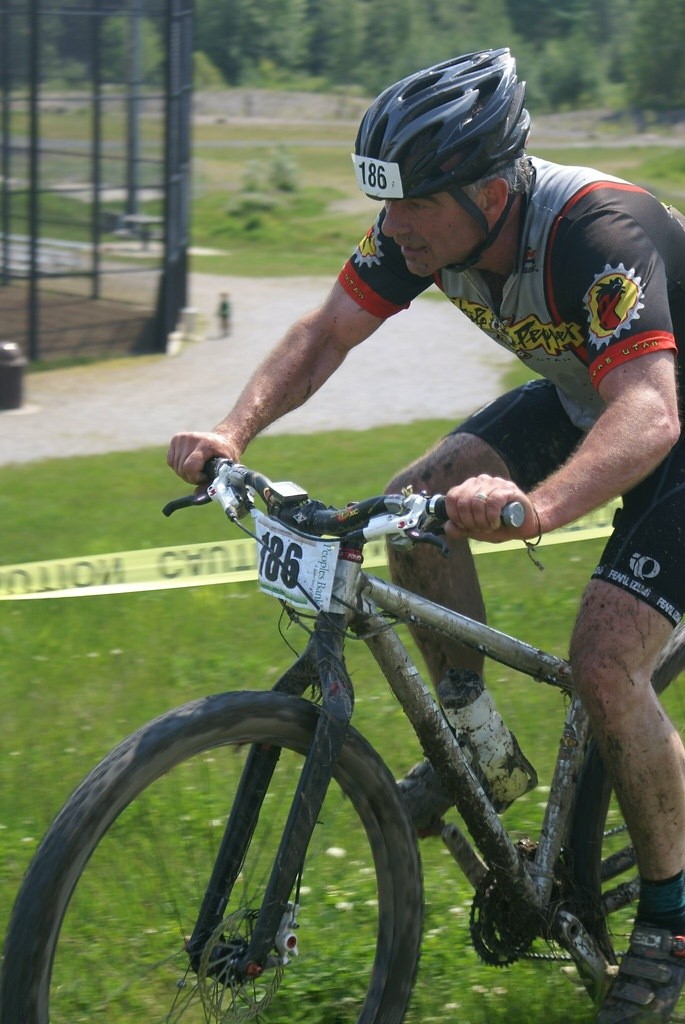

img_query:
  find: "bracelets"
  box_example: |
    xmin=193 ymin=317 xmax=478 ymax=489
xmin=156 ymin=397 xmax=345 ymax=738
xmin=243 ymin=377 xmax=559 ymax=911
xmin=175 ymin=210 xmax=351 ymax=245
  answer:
xmin=520 ymin=503 xmax=546 ymax=576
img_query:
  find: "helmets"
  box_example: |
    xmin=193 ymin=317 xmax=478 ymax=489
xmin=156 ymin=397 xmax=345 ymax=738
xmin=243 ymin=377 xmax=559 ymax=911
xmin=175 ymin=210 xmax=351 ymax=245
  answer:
xmin=350 ymin=46 xmax=531 ymax=201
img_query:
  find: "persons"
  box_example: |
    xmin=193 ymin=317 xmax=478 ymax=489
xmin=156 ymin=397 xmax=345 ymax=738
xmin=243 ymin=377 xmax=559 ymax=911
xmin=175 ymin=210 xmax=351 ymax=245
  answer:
xmin=165 ymin=43 xmax=685 ymax=1024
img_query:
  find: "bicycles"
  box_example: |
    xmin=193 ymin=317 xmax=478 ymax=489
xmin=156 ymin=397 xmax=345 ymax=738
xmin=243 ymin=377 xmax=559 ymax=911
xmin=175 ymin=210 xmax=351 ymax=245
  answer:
xmin=0 ymin=455 xmax=685 ymax=1024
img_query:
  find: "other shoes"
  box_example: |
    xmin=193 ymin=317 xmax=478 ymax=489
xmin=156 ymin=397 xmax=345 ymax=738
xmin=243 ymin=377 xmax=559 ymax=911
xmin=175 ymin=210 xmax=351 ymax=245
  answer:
xmin=592 ymin=924 xmax=685 ymax=1024
xmin=395 ymin=712 xmax=540 ymax=839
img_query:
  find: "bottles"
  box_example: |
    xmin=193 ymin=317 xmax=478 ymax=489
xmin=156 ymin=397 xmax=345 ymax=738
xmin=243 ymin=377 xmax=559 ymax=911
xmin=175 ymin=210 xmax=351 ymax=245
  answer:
xmin=436 ymin=667 xmax=528 ymax=802
